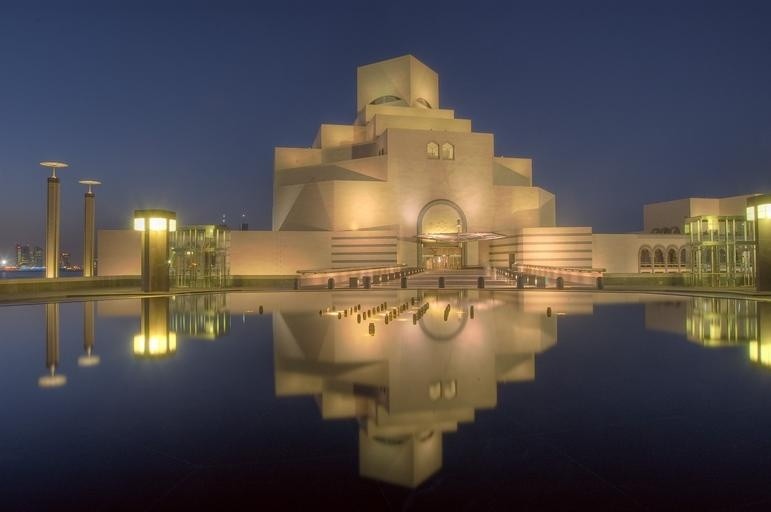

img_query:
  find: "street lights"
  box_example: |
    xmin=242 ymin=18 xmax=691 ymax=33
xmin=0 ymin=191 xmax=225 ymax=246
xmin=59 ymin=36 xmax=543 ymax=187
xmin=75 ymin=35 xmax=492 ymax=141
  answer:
xmin=39 ymin=160 xmax=69 ymax=279
xmin=79 ymin=179 xmax=102 ymax=278
xmin=77 ymin=300 xmax=101 ymax=368
xmin=38 ymin=302 xmax=68 ymax=388
